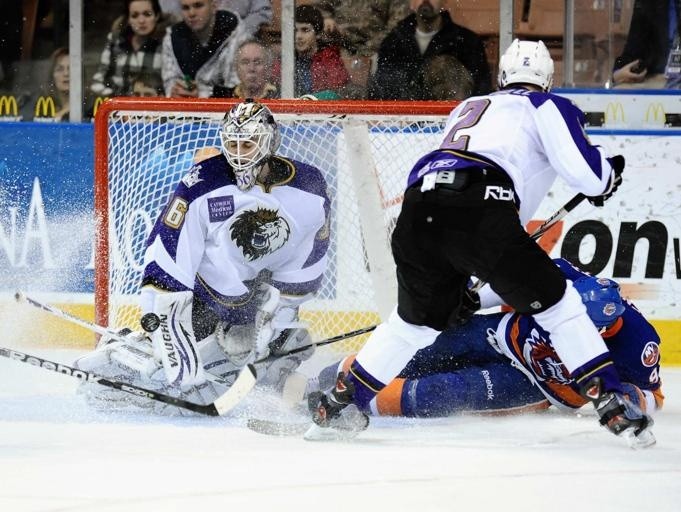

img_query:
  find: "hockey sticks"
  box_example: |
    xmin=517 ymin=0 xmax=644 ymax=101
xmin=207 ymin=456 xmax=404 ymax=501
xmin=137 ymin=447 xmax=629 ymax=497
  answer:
xmin=0 ymin=347 xmax=257 ymax=415
xmin=15 ymin=292 xmax=309 ymax=413
xmin=246 ymin=418 xmax=316 ymax=438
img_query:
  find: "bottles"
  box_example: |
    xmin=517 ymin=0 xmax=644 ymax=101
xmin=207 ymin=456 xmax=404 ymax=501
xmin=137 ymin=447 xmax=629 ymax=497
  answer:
xmin=182 ymin=73 xmax=192 ymax=92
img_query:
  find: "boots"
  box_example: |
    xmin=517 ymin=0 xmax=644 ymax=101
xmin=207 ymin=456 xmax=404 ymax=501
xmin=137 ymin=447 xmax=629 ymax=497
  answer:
xmin=313 ymin=371 xmax=369 ymax=432
xmin=581 ymin=377 xmax=653 ymax=434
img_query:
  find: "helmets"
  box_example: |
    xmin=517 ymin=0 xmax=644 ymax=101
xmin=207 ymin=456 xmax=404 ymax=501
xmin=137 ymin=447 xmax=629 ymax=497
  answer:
xmin=219 ymin=98 xmax=280 ymax=189
xmin=574 ymin=277 xmax=625 ymax=330
xmin=497 ymin=37 xmax=555 ymax=93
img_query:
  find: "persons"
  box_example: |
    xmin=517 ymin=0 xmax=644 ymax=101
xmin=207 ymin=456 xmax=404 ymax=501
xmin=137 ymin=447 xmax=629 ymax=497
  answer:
xmin=306 ymin=38 xmax=654 ymax=436
xmin=317 ymin=258 xmax=665 ymax=419
xmin=71 ymin=99 xmax=331 ymax=420
xmin=613 ymin=0 xmax=680 ymax=86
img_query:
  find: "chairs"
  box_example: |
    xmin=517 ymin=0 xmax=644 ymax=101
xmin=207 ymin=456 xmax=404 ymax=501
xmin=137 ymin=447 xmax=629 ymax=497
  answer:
xmin=258 ymin=1 xmax=372 ymax=98
xmin=444 ymin=2 xmax=633 ymax=90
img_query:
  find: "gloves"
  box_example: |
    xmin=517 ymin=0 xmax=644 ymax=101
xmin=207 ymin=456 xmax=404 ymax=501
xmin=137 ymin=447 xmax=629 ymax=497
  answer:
xmin=588 ymin=155 xmax=624 ymax=206
xmin=456 ymin=288 xmax=481 ymax=322
